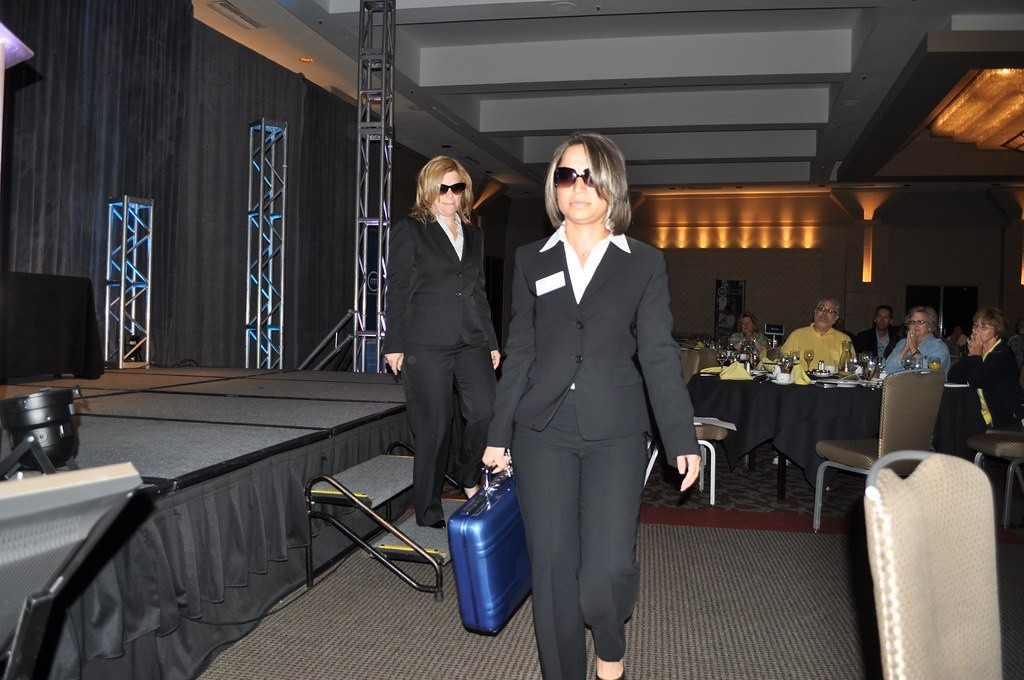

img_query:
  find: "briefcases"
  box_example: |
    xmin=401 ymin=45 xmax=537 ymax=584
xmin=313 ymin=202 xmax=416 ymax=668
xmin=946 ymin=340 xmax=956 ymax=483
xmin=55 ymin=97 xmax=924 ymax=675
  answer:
xmin=447 ymin=463 xmax=531 ymax=637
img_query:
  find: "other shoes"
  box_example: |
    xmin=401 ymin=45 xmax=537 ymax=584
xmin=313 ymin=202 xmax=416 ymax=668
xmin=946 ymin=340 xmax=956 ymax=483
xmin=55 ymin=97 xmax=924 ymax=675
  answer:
xmin=595 ymin=668 xmax=625 ymax=680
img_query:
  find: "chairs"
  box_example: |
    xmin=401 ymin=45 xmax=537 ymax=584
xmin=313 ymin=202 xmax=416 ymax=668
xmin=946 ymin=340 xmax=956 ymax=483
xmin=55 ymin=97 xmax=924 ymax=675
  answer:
xmin=644 ymin=347 xmax=728 ymax=506
xmin=863 ymin=450 xmax=1004 ymax=680
xmin=812 ymin=368 xmax=946 ymax=534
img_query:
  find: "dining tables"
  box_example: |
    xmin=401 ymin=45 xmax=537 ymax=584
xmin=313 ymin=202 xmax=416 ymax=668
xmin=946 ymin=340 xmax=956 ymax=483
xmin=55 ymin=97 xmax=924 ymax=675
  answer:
xmin=683 ymin=364 xmax=990 ymax=501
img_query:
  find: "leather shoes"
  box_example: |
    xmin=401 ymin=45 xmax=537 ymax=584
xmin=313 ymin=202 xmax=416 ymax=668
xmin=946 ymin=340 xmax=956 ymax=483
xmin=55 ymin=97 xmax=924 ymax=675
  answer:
xmin=461 ymin=485 xmax=480 ymax=500
xmin=429 ymin=520 xmax=446 ymax=528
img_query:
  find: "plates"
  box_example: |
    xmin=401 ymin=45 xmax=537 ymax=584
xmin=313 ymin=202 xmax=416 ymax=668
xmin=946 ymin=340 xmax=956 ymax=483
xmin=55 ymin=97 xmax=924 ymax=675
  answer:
xmin=834 ymin=380 xmax=861 ymax=387
xmin=750 ymin=370 xmax=770 ymax=374
xmin=813 ymin=371 xmax=836 ymax=377
xmin=771 ymin=380 xmax=794 ymax=385
xmin=860 ymin=379 xmax=881 ymax=387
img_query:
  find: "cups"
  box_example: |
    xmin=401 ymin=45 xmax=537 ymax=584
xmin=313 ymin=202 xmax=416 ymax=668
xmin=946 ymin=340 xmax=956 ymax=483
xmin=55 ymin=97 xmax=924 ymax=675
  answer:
xmin=775 ymin=373 xmax=790 ymax=382
xmin=825 ymin=365 xmax=836 ymax=373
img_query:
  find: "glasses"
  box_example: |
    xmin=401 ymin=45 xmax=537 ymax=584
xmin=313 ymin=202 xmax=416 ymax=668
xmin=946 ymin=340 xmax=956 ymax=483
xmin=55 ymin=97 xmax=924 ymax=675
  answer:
xmin=436 ymin=183 xmax=467 ymax=194
xmin=553 ymin=167 xmax=604 ymax=188
xmin=816 ymin=306 xmax=837 ymax=314
xmin=908 ymin=320 xmax=932 ymax=325
xmin=973 ymin=322 xmax=996 ymax=331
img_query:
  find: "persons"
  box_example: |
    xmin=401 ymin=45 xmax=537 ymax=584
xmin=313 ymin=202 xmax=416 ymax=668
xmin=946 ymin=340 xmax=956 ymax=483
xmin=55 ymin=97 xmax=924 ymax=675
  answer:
xmin=834 ymin=304 xmax=1024 ymax=371
xmin=772 ymin=296 xmax=857 ymax=467
xmin=929 ymin=306 xmax=1024 ymax=455
xmin=382 ymin=155 xmax=501 ymax=530
xmin=728 ymin=312 xmax=768 ymax=353
xmin=480 ymin=131 xmax=704 ymax=680
xmin=884 ymin=304 xmax=950 ymax=382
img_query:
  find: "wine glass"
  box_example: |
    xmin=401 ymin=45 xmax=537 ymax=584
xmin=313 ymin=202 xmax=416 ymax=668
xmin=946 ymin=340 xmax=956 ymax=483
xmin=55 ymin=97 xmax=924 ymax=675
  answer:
xmin=838 ymin=340 xmax=941 ymax=387
xmin=717 ymin=339 xmax=813 ymax=379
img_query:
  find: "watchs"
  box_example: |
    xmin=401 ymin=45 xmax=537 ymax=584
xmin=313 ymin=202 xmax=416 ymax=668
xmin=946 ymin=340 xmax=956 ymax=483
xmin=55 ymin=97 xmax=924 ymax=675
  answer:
xmin=912 ymin=350 xmax=920 ymax=356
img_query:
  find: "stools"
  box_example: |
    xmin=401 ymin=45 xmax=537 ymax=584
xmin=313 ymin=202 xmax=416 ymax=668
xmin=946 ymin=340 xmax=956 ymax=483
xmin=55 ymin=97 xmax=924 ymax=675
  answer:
xmin=968 ymin=428 xmax=1024 ymax=529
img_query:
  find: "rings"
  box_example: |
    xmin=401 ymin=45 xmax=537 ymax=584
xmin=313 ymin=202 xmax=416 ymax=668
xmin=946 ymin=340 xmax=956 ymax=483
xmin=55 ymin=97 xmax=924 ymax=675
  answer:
xmin=910 ymin=335 xmax=913 ymax=337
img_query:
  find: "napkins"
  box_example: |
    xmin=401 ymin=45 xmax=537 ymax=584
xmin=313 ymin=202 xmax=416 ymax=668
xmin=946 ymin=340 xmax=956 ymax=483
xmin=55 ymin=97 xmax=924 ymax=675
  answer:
xmin=699 ymin=366 xmax=728 ymax=374
xmin=719 ymin=361 xmax=752 ymax=380
xmin=757 ymin=357 xmax=774 ymax=371
xmin=790 ymin=365 xmax=812 ymax=385
